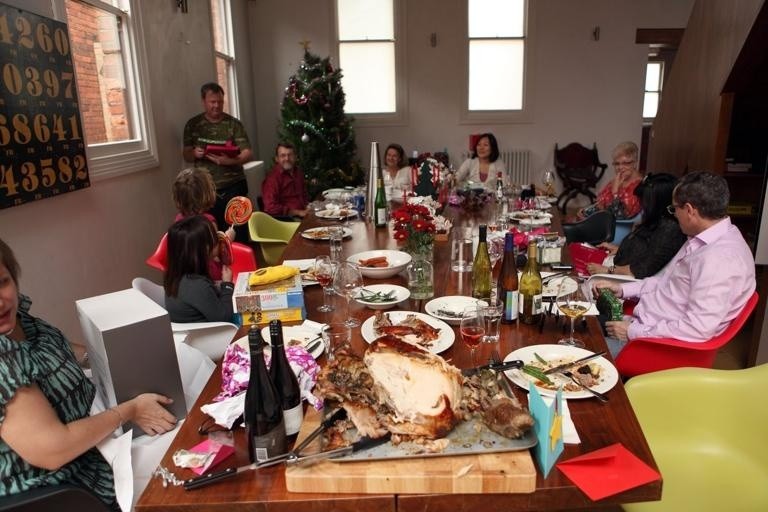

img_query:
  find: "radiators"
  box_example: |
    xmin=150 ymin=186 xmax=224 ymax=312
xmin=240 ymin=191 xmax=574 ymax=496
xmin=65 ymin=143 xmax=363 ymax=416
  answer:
xmin=464 ymin=149 xmax=532 ymax=185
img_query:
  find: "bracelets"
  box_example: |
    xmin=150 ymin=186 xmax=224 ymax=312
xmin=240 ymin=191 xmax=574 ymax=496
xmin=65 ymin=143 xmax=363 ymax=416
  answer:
xmin=110 ymin=407 xmax=122 ymax=432
xmin=81 ymin=352 xmax=88 ymax=368
xmin=608 ymin=265 xmax=616 ymax=273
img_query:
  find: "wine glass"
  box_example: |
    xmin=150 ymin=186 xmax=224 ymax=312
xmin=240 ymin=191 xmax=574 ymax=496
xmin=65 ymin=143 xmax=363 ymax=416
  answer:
xmin=314 ymin=253 xmax=367 ymax=328
xmin=460 ymin=306 xmax=485 ymax=366
xmin=542 ymin=172 xmax=557 ymax=199
xmin=557 ymin=276 xmax=594 ymax=347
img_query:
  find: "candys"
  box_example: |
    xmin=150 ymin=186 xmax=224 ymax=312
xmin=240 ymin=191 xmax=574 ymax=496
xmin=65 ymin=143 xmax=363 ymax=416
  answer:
xmin=224 ymin=195 xmax=253 ymax=226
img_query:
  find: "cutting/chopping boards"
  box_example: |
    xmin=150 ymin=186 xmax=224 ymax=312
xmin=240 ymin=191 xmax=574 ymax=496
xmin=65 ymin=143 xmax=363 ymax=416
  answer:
xmin=287 ymin=382 xmax=539 ymax=495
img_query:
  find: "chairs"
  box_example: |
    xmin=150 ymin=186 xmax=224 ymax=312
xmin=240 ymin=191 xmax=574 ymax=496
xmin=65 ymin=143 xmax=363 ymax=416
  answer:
xmin=248 ymin=210 xmax=301 ymax=266
xmin=146 ymin=230 xmax=257 ymax=288
xmin=554 ymin=142 xmax=608 ymax=215
xmin=132 ymin=277 xmax=239 ymax=361
xmin=561 ymin=210 xmax=615 ymax=245
xmin=619 ymin=362 xmax=768 ymax=512
xmin=612 ymin=291 xmax=760 ymax=375
xmin=609 ymin=210 xmax=643 ymax=245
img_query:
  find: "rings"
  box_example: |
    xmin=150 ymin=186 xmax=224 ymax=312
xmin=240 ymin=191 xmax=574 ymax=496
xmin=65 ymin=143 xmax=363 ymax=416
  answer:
xmin=590 ymin=265 xmax=592 ymax=268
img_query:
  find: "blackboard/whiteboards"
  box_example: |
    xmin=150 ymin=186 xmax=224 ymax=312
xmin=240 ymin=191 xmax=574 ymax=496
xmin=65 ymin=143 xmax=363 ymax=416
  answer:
xmin=0 ymin=2 xmax=91 ymax=210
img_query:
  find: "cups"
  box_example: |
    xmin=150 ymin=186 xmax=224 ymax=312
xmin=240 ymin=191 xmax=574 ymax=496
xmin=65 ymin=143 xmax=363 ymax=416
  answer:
xmin=452 ymin=228 xmax=472 ymax=271
xmin=488 ymin=213 xmax=509 ymax=232
xmin=477 ymin=299 xmax=502 ymax=342
xmin=328 ymin=186 xmax=363 ymax=251
xmin=322 ymin=324 xmax=352 ymax=366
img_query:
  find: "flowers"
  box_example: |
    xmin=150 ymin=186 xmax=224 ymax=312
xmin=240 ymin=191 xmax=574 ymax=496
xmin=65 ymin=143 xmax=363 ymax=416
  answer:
xmin=386 ymin=201 xmax=435 ymax=293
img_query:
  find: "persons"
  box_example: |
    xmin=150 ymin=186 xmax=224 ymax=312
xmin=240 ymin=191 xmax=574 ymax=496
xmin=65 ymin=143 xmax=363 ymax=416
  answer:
xmin=261 ymin=141 xmax=311 ymax=223
xmin=0 ymin=240 xmax=178 ymax=511
xmin=382 ymin=143 xmax=419 ymax=195
xmin=183 ymin=83 xmax=253 ymax=244
xmin=568 ymin=172 xmax=687 ymax=284
xmin=162 ymin=214 xmax=242 ymax=328
xmin=448 ymin=133 xmax=513 ymax=190
xmin=561 ymin=141 xmax=648 ymax=247
xmin=591 ymin=170 xmax=758 ymax=358
xmin=171 ymin=166 xmax=238 ymax=281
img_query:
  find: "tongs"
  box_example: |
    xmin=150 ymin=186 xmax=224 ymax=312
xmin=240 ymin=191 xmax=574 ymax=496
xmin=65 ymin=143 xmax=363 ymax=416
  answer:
xmin=283 ymin=407 xmax=391 ymax=468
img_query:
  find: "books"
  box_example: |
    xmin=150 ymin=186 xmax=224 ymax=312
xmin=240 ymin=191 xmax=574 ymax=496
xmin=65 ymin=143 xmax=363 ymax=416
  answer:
xmin=201 ymin=145 xmax=241 ymax=160
xmin=725 ymin=156 xmax=753 ymax=173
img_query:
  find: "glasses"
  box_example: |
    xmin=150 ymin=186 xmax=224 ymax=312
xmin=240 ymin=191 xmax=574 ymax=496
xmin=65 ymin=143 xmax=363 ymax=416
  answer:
xmin=667 ymin=202 xmax=689 ymax=215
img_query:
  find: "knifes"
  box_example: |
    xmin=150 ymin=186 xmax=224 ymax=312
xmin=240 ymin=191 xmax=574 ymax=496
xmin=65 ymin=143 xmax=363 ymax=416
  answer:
xmin=461 ymin=360 xmax=524 ymax=377
xmin=183 ymin=453 xmax=291 ymax=489
xmin=544 ymin=348 xmax=606 ymax=376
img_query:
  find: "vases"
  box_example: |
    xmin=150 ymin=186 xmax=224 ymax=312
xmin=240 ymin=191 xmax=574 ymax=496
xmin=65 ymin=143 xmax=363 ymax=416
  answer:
xmin=407 ymin=249 xmax=434 ymax=298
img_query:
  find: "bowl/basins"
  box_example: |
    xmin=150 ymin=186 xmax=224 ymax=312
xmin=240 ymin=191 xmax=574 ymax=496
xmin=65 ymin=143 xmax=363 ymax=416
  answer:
xmin=346 ymin=249 xmax=410 ymax=279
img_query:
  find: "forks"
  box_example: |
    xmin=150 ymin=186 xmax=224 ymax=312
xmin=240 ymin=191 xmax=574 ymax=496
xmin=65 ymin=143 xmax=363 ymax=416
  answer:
xmin=558 ymin=369 xmax=615 ymax=404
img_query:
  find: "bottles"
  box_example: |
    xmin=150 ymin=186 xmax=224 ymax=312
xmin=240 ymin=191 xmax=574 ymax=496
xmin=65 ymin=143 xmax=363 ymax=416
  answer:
xmin=363 ymin=139 xmax=389 ymax=230
xmin=472 ymin=223 xmax=544 ymax=325
xmin=494 ymin=171 xmax=521 ymax=204
xmin=245 ymin=319 xmax=303 ymax=468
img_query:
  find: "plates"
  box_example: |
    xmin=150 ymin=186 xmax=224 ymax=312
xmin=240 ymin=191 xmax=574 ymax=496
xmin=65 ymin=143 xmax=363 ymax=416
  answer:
xmin=322 ymin=187 xmax=344 ymax=200
xmin=513 ymin=273 xmax=583 ymax=296
xmin=362 ymin=177 xmax=417 ymax=201
xmin=277 ymin=331 xmax=325 ymax=361
xmin=510 ymin=195 xmax=555 ymax=223
xmin=301 ymin=225 xmax=353 ymax=241
xmin=282 ymin=258 xmax=319 ymax=287
xmin=352 ymin=284 xmax=489 ymax=357
xmin=317 ymin=207 xmax=357 ymax=219
xmin=503 ymin=342 xmax=621 ymax=398
